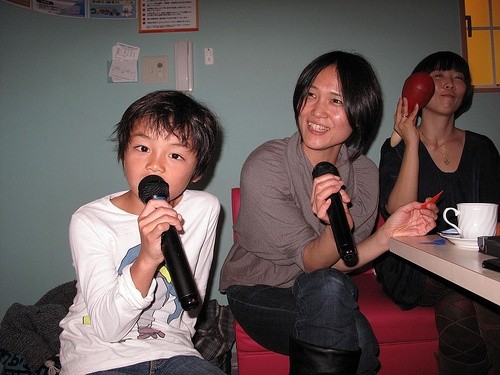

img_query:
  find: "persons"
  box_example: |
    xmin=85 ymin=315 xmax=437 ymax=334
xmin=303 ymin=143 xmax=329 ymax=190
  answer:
xmin=59 ymin=89 xmax=225 ymax=374
xmin=218 ymin=51 xmax=439 ymax=375
xmin=373 ymin=49 xmax=500 ymax=375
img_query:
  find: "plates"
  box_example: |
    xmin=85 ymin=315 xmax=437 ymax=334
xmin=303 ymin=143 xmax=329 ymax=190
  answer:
xmin=438 ymin=228 xmax=480 ymax=251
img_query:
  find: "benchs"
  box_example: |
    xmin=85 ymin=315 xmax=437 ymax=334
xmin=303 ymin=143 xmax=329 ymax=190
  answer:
xmin=229 ymin=184 xmax=442 ymax=375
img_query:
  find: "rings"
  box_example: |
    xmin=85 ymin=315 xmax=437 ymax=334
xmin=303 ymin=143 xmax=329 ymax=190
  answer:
xmin=403 ymin=116 xmax=409 ymax=117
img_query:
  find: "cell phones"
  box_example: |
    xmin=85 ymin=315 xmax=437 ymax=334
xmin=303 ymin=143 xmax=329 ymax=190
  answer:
xmin=482 ymin=257 xmax=500 ymax=272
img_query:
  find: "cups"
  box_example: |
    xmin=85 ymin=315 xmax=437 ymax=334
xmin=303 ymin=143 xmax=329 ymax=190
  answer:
xmin=443 ymin=203 xmax=498 ymax=239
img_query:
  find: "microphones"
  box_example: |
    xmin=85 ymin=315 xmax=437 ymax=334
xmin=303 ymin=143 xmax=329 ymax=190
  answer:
xmin=311 ymin=161 xmax=357 ymax=261
xmin=138 ymin=175 xmax=200 ymax=311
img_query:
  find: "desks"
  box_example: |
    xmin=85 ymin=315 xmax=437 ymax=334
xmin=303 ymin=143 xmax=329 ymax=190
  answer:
xmin=390 ymin=228 xmax=500 ymax=306
